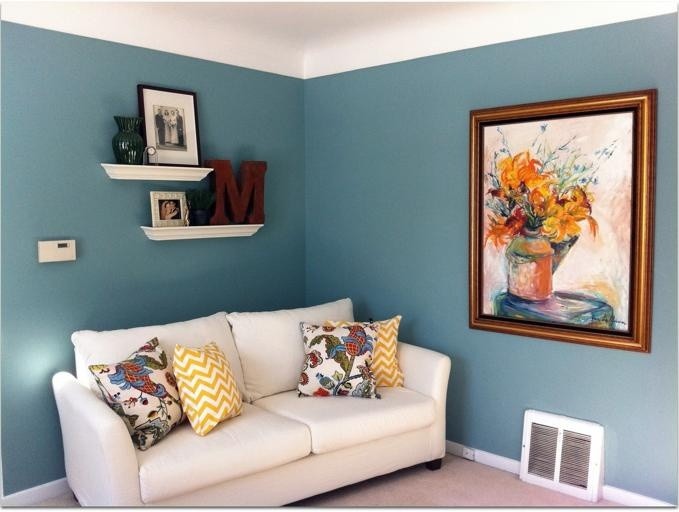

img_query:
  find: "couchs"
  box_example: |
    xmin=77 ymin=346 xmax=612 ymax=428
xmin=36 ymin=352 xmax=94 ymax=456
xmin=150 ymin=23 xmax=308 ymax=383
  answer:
xmin=51 ymin=341 xmax=452 ymax=506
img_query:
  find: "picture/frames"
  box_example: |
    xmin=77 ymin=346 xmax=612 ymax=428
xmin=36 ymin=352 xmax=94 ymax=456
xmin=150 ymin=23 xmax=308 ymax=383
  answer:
xmin=468 ymin=89 xmax=657 ymax=354
xmin=134 ymin=84 xmax=202 ymax=166
xmin=149 ymin=191 xmax=191 ymax=227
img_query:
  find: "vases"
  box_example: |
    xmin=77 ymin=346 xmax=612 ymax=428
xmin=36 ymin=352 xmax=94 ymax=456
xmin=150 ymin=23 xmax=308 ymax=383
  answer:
xmin=111 ymin=115 xmax=144 ymax=164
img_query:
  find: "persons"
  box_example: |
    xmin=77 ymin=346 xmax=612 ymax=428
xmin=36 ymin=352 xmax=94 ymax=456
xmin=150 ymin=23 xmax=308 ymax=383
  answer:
xmin=175 ymin=108 xmax=185 ymax=146
xmin=161 ymin=201 xmax=180 ymax=220
xmin=162 ymin=109 xmax=172 ymax=144
xmin=155 ymin=106 xmax=165 ymax=145
xmin=170 ymin=110 xmax=179 ymax=145
xmin=169 ymin=200 xmax=181 ymax=219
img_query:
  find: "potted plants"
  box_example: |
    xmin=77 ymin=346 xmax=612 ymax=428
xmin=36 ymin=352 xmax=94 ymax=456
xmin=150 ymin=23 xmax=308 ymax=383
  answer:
xmin=186 ymin=188 xmax=214 ymax=225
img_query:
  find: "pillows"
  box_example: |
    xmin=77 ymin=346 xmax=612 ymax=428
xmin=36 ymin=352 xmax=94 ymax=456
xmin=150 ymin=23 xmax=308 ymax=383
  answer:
xmin=169 ymin=341 xmax=244 ymax=438
xmin=297 ymin=321 xmax=383 ymax=401
xmin=324 ymin=314 xmax=405 ymax=388
xmin=87 ymin=336 xmax=188 ymax=448
